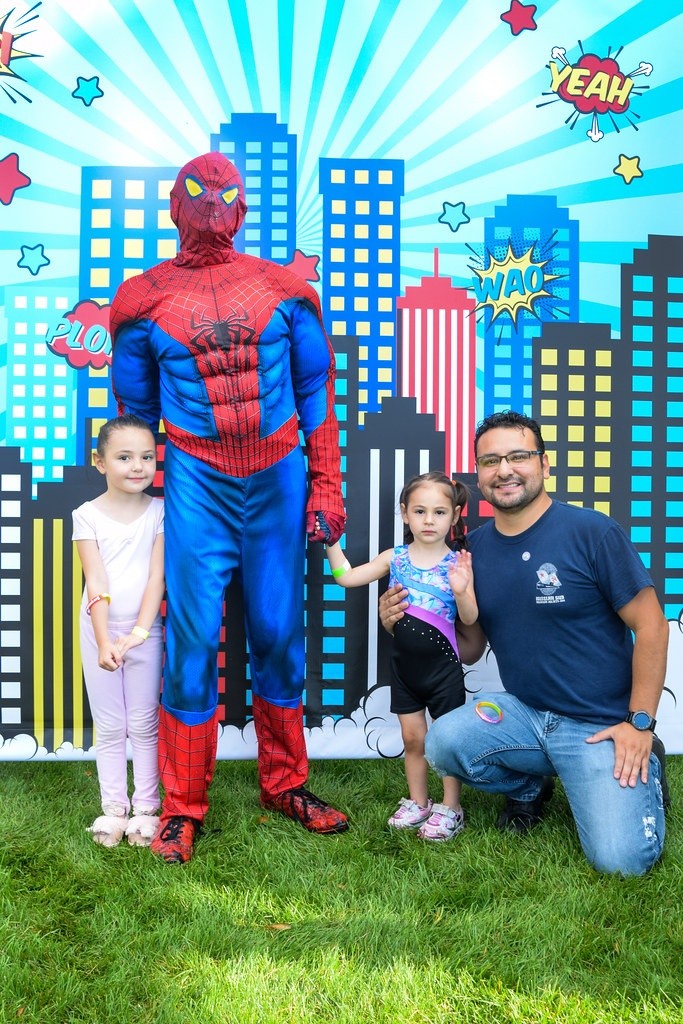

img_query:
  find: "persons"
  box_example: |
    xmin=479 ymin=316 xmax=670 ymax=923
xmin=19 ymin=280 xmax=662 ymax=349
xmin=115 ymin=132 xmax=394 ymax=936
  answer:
xmin=315 ymin=470 xmax=479 ymax=842
xmin=379 ymin=413 xmax=670 ymax=878
xmin=108 ymin=153 xmax=347 ymax=865
xmin=72 ymin=416 xmax=166 ymax=847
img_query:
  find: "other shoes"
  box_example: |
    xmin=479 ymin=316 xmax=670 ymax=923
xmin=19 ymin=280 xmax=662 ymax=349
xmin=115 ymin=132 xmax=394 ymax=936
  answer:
xmin=126 ymin=814 xmax=158 ymax=847
xmin=85 ymin=815 xmax=128 ymax=848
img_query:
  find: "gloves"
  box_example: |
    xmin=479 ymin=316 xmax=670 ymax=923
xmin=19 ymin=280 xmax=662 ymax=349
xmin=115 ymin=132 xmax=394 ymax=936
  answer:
xmin=307 ymin=510 xmax=345 ymax=546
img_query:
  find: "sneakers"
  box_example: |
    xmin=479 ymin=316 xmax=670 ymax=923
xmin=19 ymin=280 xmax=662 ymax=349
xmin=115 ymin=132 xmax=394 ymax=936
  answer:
xmin=387 ymin=798 xmax=433 ymax=829
xmin=149 ymin=816 xmax=199 ymax=862
xmin=265 ymin=785 xmax=348 ymax=832
xmin=495 ymin=776 xmax=557 ymax=831
xmin=651 ymin=735 xmax=672 ymax=814
xmin=417 ymin=803 xmax=466 ymax=842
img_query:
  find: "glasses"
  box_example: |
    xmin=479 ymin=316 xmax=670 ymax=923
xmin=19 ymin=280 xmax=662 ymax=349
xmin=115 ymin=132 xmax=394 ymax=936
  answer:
xmin=474 ymin=451 xmax=542 ymax=468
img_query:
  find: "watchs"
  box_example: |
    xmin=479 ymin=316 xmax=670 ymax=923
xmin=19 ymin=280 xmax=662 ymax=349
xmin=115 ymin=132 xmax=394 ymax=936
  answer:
xmin=625 ymin=710 xmax=657 ymax=732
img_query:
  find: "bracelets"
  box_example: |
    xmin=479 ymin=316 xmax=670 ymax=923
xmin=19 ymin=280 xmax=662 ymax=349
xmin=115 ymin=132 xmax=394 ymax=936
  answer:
xmin=86 ymin=593 xmax=110 ymax=616
xmin=132 ymin=626 xmax=150 ymax=639
xmin=331 ymin=559 xmax=350 ymax=578
